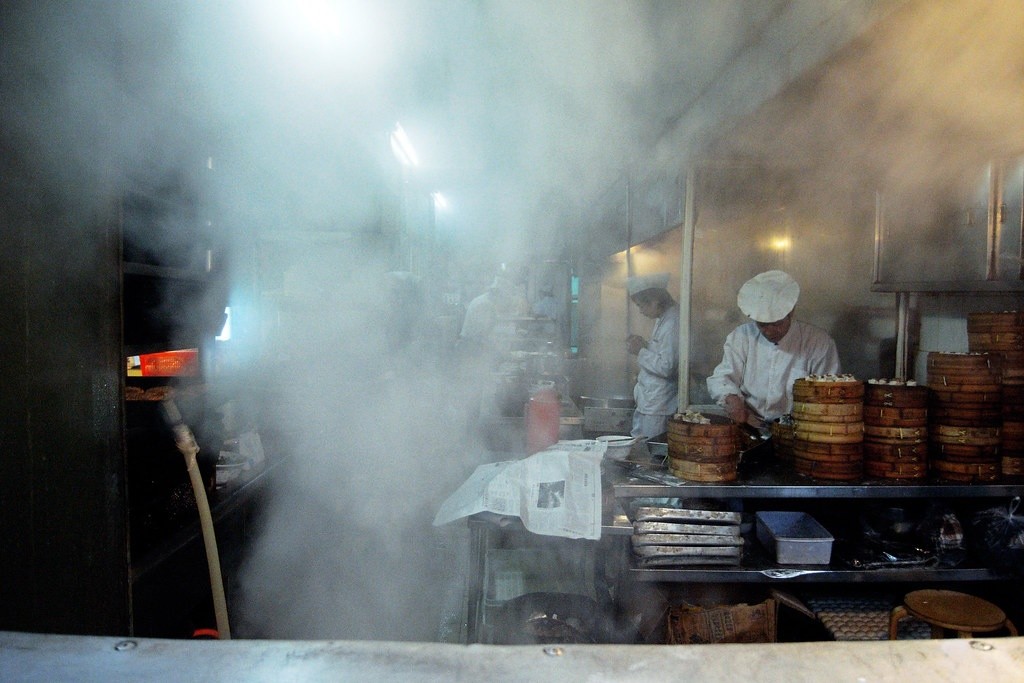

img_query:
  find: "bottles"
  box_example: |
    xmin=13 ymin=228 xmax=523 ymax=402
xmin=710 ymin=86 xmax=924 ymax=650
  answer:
xmin=520 ymin=384 xmax=560 ymax=456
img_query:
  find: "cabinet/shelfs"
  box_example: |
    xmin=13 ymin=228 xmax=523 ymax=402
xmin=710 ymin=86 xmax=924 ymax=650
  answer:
xmin=597 ymin=457 xmax=1024 ymax=644
xmin=871 ymin=137 xmax=1024 ymax=292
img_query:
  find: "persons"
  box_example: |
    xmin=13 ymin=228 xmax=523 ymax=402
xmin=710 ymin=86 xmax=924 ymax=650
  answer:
xmin=705 ymin=270 xmax=841 ymax=439
xmin=460 ymin=263 xmax=561 ymax=344
xmin=624 ymin=273 xmax=680 ymax=438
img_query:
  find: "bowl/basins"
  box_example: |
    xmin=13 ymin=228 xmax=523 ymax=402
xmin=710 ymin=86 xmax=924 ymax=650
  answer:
xmin=213 ymin=450 xmax=248 ymax=490
xmin=595 ymin=434 xmax=635 ymax=466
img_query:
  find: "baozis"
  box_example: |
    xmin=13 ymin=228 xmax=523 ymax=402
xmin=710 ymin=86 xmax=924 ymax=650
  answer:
xmin=869 ymin=377 xmax=916 ymax=386
xmin=674 ymin=410 xmax=710 ymax=424
xmin=808 ymin=600 xmax=931 ymax=642
xmin=805 ymin=374 xmax=856 ymax=382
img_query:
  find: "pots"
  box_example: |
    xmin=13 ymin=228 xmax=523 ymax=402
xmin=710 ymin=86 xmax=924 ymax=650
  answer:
xmin=568 ymin=393 xmax=637 ymax=419
xmin=580 ymin=392 xmax=635 ymax=408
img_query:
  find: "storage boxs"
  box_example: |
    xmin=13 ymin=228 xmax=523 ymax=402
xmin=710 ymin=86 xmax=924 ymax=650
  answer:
xmin=753 ymin=510 xmax=835 ymax=567
xmin=662 ymin=594 xmax=776 ymax=645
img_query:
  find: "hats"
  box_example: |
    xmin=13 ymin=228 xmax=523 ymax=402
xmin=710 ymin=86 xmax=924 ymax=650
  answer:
xmin=539 ymin=281 xmax=553 ymax=292
xmin=621 ymin=271 xmax=672 ymax=298
xmin=490 ymin=276 xmax=511 ymax=291
xmin=737 ymin=270 xmax=799 ymax=322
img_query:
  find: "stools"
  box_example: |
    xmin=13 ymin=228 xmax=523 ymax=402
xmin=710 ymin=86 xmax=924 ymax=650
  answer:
xmin=887 ymin=588 xmax=1015 ymax=641
